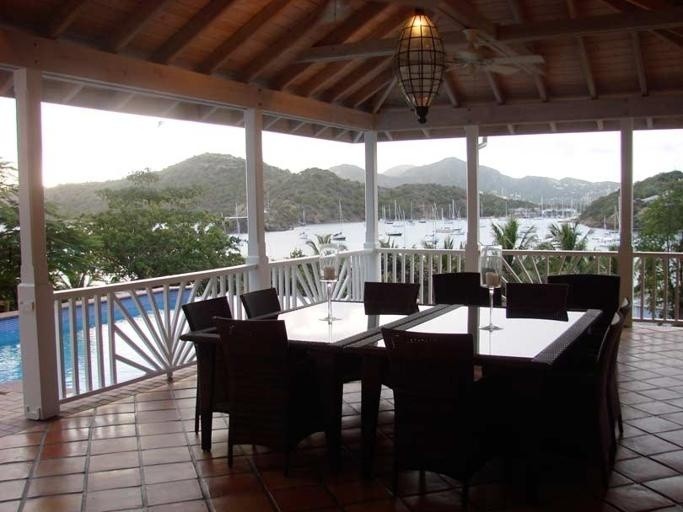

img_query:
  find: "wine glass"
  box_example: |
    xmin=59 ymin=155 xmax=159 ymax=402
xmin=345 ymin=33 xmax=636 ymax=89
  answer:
xmin=318 ymin=244 xmax=341 ymax=325
xmin=480 ymin=246 xmax=503 ymax=332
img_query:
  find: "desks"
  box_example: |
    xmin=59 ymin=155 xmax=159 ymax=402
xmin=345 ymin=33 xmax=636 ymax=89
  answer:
xmin=341 ymin=305 xmax=602 ymax=489
xmin=179 ymin=300 xmax=448 ymax=463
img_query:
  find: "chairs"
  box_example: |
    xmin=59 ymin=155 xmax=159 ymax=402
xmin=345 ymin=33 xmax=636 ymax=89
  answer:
xmin=179 ymin=296 xmax=236 ymax=431
xmin=506 ymin=282 xmax=569 ymax=309
xmin=564 ymin=298 xmax=628 ymax=436
xmin=528 ymin=315 xmax=624 ymax=487
xmin=214 ymin=320 xmax=306 ymax=476
xmin=362 ymin=281 xmax=418 ymax=307
xmin=382 ymin=326 xmax=510 ymax=511
xmin=240 ymin=288 xmax=281 ymax=316
xmin=432 ymin=271 xmax=480 ymax=307
xmin=544 ymin=272 xmax=626 ymax=304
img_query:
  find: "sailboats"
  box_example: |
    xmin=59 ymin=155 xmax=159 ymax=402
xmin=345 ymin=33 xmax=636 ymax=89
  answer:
xmin=379 ymin=199 xmax=466 ymax=236
xmin=298 ymin=209 xmax=308 ymax=240
xmin=500 ymin=194 xmax=584 ymax=223
xmin=592 ymin=197 xmax=621 ymax=247
xmin=332 ymin=199 xmax=346 ymax=240
xmin=221 ymin=203 xmax=248 ymax=246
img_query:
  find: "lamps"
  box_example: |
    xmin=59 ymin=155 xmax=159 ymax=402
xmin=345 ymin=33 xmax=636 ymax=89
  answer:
xmin=393 ymin=0 xmax=444 ymax=124
